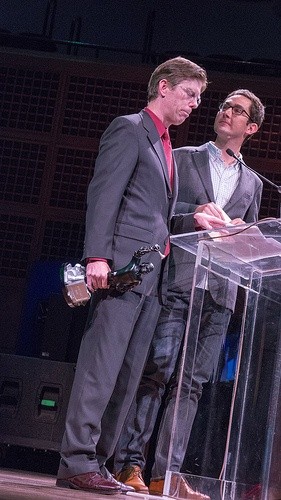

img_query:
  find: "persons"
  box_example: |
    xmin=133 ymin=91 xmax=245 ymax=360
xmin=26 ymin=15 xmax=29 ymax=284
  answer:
xmin=56 ymin=56 xmax=231 ymax=494
xmin=114 ymin=89 xmax=263 ymax=500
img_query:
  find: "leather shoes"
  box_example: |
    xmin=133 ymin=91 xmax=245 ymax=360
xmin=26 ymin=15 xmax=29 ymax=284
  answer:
xmin=148 ymin=474 xmax=212 ymax=500
xmin=57 ymin=471 xmax=122 ymax=494
xmin=99 ymin=471 xmax=135 ymax=493
xmin=114 ymin=465 xmax=150 ymax=494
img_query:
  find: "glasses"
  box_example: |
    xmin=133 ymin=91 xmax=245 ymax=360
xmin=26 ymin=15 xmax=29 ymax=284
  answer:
xmin=218 ymin=102 xmax=253 ymax=122
xmin=179 ymin=85 xmax=202 ymax=107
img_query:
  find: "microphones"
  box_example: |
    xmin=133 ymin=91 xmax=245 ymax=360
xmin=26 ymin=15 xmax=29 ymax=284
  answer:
xmin=226 ymin=148 xmax=281 ymax=193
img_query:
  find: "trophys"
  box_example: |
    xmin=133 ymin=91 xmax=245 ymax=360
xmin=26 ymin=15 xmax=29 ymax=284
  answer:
xmin=60 ymin=244 xmax=165 ymax=308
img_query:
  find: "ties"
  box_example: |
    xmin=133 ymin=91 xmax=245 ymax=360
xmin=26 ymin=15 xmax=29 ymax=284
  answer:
xmin=161 ymin=127 xmax=174 ymax=197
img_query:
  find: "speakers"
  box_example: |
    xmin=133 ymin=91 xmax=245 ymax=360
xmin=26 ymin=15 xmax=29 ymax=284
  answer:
xmin=0 ymin=352 xmax=77 ymax=474
